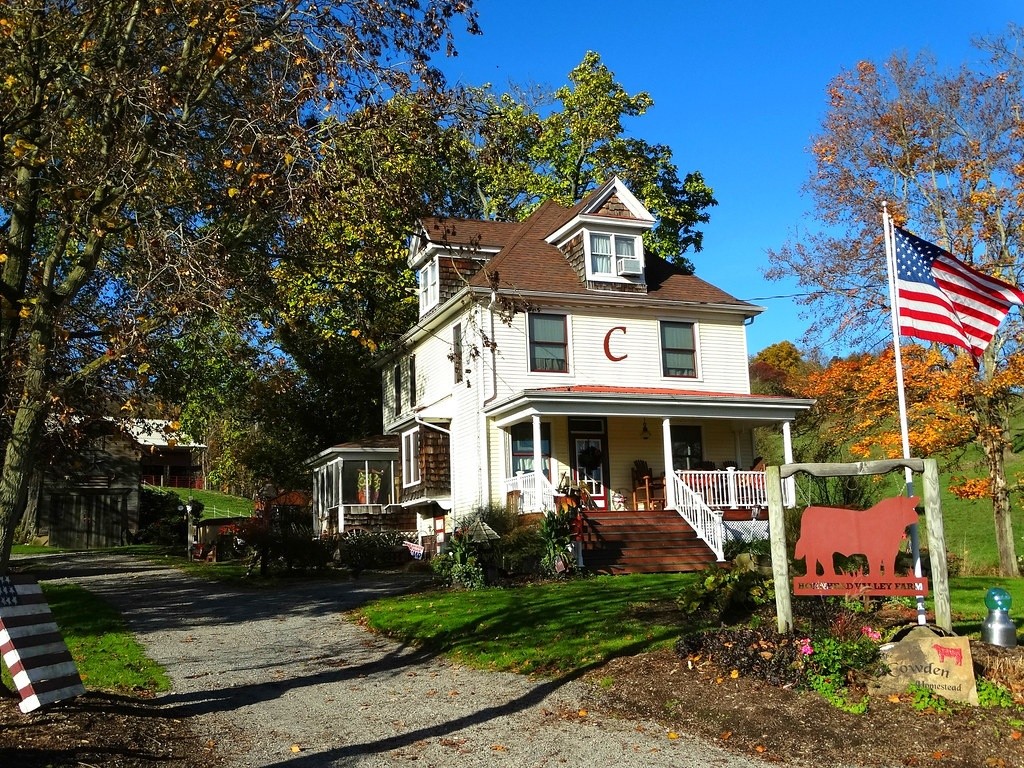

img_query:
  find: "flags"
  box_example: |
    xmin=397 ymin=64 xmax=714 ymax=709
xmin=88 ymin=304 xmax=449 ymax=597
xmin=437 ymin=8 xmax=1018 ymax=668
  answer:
xmin=893 ymin=225 xmax=1024 ymax=357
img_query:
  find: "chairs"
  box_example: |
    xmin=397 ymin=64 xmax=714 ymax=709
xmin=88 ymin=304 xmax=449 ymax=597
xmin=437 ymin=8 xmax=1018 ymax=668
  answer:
xmin=631 ymin=456 xmax=767 ymax=510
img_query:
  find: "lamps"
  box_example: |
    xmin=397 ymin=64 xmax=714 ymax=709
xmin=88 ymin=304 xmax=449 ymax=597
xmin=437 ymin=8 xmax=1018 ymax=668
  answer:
xmin=640 ymin=416 xmax=651 ymax=439
xmin=981 ymin=587 xmax=1017 ymax=647
